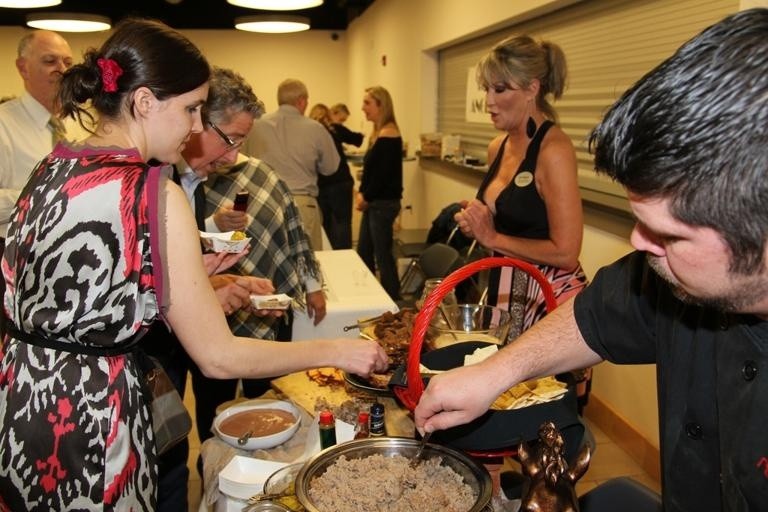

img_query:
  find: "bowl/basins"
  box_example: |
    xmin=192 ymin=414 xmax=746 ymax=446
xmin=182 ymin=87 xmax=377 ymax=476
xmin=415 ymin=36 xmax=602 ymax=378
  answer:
xmin=213 ymin=399 xmax=302 ymax=452
xmin=295 ymin=436 xmax=494 ymax=512
xmin=425 ymin=302 xmax=512 ymax=346
xmin=262 ymin=464 xmax=305 ymax=508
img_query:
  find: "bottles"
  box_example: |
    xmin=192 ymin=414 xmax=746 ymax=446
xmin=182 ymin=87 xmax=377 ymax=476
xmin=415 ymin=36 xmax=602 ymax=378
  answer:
xmin=317 ymin=402 xmax=387 ymax=448
xmin=419 ymin=276 xmax=459 ymax=326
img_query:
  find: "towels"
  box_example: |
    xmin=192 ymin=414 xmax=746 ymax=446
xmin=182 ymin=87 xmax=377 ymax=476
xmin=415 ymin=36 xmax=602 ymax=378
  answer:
xmin=200 ymin=401 xmax=314 ymax=505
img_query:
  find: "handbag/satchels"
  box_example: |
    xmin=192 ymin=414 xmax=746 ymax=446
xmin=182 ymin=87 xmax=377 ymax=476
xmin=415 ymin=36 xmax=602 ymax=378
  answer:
xmin=144 ymin=357 xmax=192 ymax=457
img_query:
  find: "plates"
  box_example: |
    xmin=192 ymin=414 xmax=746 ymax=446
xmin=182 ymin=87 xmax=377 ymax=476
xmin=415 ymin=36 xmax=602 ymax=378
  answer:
xmin=340 ymin=369 xmax=391 ymax=395
xmin=215 ymin=455 xmax=288 ymax=502
xmin=248 ymin=294 xmax=295 ymax=312
xmin=197 ymin=230 xmax=252 ymax=255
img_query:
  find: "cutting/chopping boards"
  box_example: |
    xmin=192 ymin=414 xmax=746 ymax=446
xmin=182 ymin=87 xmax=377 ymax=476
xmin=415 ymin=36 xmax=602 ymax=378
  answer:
xmin=269 ymin=366 xmax=415 ymax=439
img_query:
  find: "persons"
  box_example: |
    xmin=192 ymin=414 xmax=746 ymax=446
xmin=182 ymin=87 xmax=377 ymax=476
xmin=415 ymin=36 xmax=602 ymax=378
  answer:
xmin=455 ymin=35 xmax=592 ymax=414
xmin=243 ymin=80 xmax=340 ymax=252
xmin=191 ymin=152 xmax=326 ymax=478
xmin=308 ymin=104 xmax=363 ymax=251
xmin=0 ymin=29 xmax=93 ymax=257
xmin=2 ymin=19 xmax=393 ymax=510
xmin=357 ymin=86 xmax=403 ymax=302
xmin=332 ymin=104 xmax=351 ymax=122
xmin=415 ymin=9 xmax=768 ymax=511
xmin=141 ymin=67 xmax=272 ymax=511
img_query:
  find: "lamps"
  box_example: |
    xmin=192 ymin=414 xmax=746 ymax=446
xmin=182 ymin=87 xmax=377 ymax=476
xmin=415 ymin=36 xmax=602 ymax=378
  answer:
xmin=24 ymin=12 xmax=112 ymax=33
xmin=233 ymin=13 xmax=311 ymax=34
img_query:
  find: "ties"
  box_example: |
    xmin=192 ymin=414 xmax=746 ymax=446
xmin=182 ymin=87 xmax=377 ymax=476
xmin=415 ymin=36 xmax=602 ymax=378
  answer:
xmin=50 ymin=118 xmax=70 ymax=147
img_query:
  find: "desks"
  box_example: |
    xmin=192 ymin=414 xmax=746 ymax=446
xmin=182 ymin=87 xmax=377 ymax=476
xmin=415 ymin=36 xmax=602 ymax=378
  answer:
xmin=295 ymin=248 xmax=400 ymax=344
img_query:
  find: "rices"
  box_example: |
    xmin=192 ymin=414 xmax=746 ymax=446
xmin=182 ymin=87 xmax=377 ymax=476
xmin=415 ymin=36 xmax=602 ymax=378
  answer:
xmin=308 ymin=453 xmax=477 ymax=512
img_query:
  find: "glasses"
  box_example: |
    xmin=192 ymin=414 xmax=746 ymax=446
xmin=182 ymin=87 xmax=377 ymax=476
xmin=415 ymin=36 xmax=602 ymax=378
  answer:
xmin=201 ymin=116 xmax=245 ymax=150
